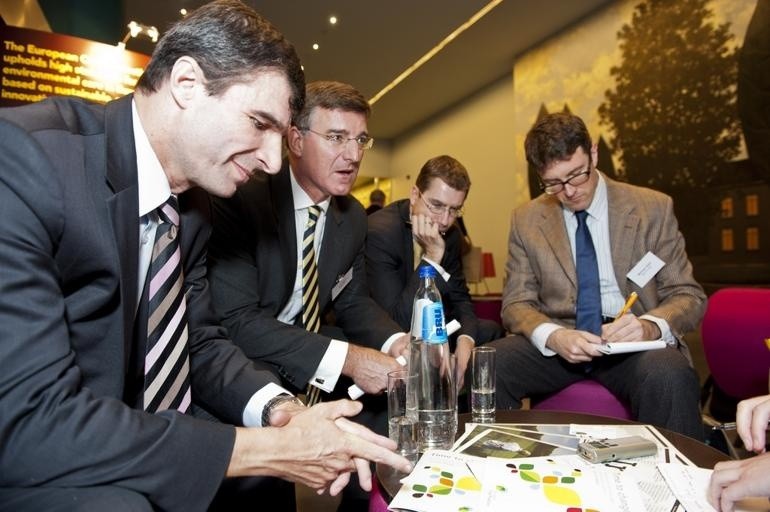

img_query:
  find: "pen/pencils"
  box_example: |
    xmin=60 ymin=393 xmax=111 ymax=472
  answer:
xmin=601 ymin=291 xmax=639 ymax=345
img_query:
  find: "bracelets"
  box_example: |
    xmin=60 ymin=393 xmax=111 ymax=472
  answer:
xmin=262 ymin=394 xmax=299 ymax=427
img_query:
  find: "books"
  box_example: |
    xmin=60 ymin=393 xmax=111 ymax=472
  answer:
xmin=590 ymin=339 xmax=669 ymax=357
xmin=344 ymin=318 xmax=461 ymax=400
xmin=385 ymin=421 xmax=715 ymax=511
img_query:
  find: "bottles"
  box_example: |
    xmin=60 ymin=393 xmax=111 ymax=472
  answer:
xmin=406 ymin=262 xmax=458 ymax=452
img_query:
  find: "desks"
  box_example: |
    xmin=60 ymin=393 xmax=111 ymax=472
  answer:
xmin=367 ymin=407 xmax=770 ymax=512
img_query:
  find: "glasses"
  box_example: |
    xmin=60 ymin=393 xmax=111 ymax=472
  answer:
xmin=537 ymin=155 xmax=591 ymax=196
xmin=420 ymin=192 xmax=464 ymax=218
xmin=298 ymin=127 xmax=373 ymax=150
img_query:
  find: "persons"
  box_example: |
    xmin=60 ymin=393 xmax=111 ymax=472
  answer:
xmin=366 ymin=155 xmax=502 ymax=411
xmin=706 ymin=393 xmax=770 ymax=511
xmin=365 ymin=189 xmax=385 ymax=216
xmin=206 ymin=79 xmax=450 ymax=511
xmin=0 ymin=0 xmax=414 ymax=511
xmin=462 ymin=112 xmax=708 ymax=444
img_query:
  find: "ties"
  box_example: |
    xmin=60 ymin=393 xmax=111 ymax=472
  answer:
xmin=301 ymin=206 xmax=322 ymax=406
xmin=142 ymin=193 xmax=194 ymax=418
xmin=575 ymin=210 xmax=602 ymax=373
xmin=414 ymin=243 xmax=424 ymax=271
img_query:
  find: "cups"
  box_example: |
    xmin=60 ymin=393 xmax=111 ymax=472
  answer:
xmin=470 ymin=345 xmax=499 ymax=416
xmin=384 ymin=370 xmax=421 ymax=457
